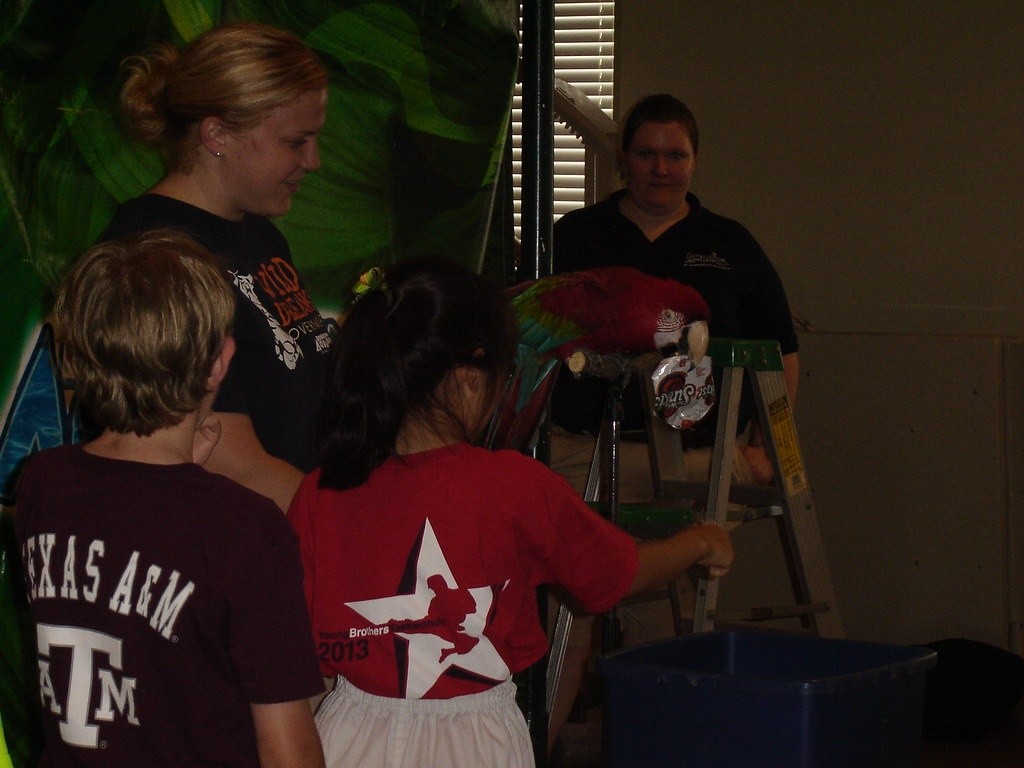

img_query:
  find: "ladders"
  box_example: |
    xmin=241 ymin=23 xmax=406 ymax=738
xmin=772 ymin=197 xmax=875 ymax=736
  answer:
xmin=547 ymin=337 xmax=846 ymax=750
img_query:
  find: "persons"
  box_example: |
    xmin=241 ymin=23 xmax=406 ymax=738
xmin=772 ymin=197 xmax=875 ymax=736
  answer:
xmin=547 ymin=93 xmax=799 ymax=748
xmin=0 ymin=229 xmax=326 ymax=768
xmin=287 ymin=256 xmax=733 ymax=768
xmin=94 ymin=21 xmax=331 ymax=515
